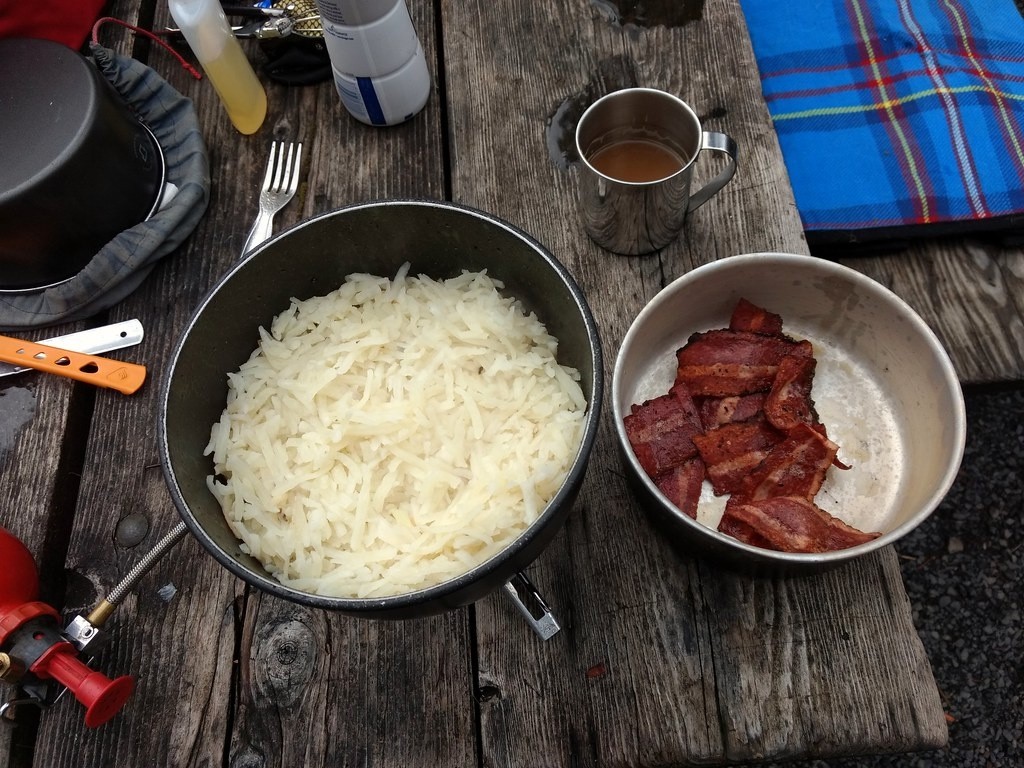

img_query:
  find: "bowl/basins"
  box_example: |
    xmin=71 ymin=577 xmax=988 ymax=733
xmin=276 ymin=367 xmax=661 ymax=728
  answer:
xmin=612 ymin=253 xmax=968 ymax=565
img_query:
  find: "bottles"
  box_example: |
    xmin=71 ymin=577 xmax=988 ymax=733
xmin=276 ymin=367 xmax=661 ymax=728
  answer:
xmin=168 ymin=0 xmax=268 ymax=135
xmin=315 ymin=0 xmax=430 ymax=127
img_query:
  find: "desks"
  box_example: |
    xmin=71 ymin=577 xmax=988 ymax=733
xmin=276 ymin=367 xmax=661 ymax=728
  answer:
xmin=0 ymin=0 xmax=946 ymax=768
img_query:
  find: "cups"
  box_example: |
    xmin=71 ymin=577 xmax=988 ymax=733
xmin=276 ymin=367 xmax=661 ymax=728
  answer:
xmin=574 ymin=87 xmax=738 ymax=255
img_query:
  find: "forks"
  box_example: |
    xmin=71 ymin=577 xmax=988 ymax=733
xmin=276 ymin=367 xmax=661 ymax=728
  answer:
xmin=240 ymin=141 xmax=302 ymax=257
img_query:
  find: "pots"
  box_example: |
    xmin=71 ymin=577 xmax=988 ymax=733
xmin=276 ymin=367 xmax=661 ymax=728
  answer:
xmin=155 ymin=200 xmax=606 ymax=620
xmin=0 ymin=35 xmax=165 ymax=294
